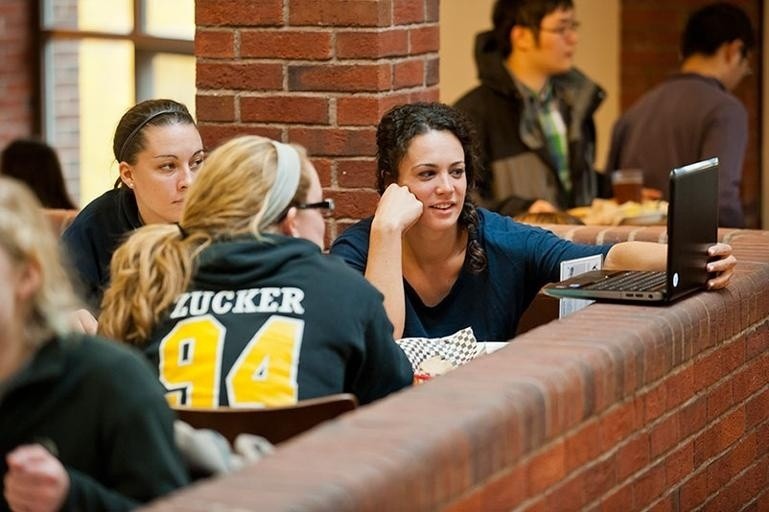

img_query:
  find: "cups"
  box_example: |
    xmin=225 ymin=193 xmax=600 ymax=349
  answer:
xmin=612 ymin=169 xmax=644 ymax=206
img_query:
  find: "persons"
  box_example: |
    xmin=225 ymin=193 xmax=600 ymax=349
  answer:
xmin=0 ymin=134 xmax=78 ymax=210
xmin=59 ymin=97 xmax=205 ymax=340
xmin=98 ymin=135 xmax=417 ymax=412
xmin=0 ymin=184 xmax=195 ymax=512
xmin=602 ymin=0 xmax=757 ymax=230
xmin=328 ymin=103 xmax=736 ymax=382
xmin=448 ymin=0 xmax=667 ymax=227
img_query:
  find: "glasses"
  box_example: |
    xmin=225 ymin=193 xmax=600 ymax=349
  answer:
xmin=298 ymin=198 xmax=335 ymax=210
xmin=540 ymin=21 xmax=579 ymax=33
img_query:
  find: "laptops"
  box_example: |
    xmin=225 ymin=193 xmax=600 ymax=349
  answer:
xmin=541 ymin=155 xmax=720 ymax=308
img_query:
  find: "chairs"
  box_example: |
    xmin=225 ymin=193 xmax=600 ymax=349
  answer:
xmin=170 ymin=393 xmax=358 ymax=454
